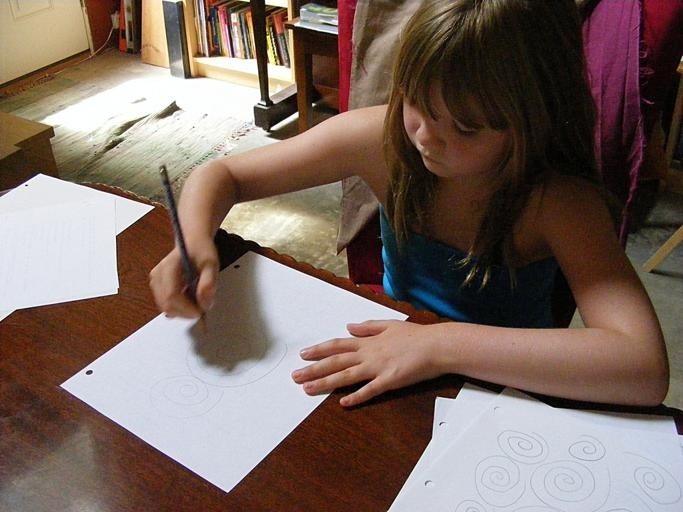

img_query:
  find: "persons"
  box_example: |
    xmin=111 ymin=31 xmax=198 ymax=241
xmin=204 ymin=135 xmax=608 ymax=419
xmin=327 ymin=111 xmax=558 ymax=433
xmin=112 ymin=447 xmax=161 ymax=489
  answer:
xmin=148 ymin=0 xmax=670 ymax=409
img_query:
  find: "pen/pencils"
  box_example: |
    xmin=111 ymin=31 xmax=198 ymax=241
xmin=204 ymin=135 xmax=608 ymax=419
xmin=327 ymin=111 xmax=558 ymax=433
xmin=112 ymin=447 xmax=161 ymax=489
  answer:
xmin=160 ymin=165 xmax=208 ymax=335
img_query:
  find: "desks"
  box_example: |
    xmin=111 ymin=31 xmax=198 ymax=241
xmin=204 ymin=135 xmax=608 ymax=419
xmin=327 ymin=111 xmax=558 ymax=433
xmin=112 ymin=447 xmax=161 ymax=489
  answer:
xmin=0 ymin=114 xmax=59 ymax=193
xmin=0 ymin=177 xmax=682 ymax=511
xmin=285 ymin=16 xmax=341 ymax=133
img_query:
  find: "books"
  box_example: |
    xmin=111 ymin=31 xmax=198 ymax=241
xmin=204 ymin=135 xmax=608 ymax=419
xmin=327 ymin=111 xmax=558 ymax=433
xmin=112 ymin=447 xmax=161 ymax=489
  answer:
xmin=192 ymin=0 xmax=291 ymax=68
